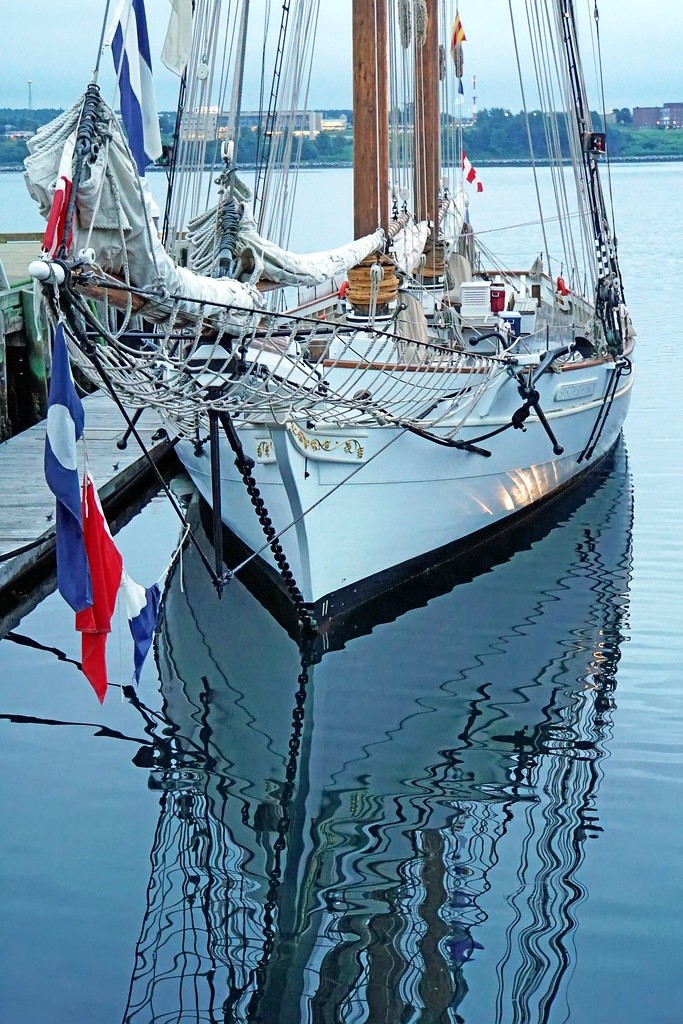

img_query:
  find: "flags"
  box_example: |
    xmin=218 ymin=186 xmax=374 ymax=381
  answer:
xmin=160 ymin=0 xmax=195 ymax=74
xmin=43 ymin=322 xmax=160 ymax=704
xmin=452 ymin=15 xmax=468 ymax=58
xmin=461 ymin=152 xmax=482 ymax=192
xmin=110 ymin=0 xmax=163 ymax=176
xmin=43 ymin=134 xmax=73 ymax=256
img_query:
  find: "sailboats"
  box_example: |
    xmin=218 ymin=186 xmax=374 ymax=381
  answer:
xmin=124 ymin=435 xmax=630 ymax=1022
xmin=33 ymin=0 xmax=634 ymax=632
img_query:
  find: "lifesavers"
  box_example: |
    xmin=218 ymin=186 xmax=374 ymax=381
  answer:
xmin=339 ymin=280 xmax=350 ymax=300
xmin=556 ymin=276 xmax=568 ymax=303
